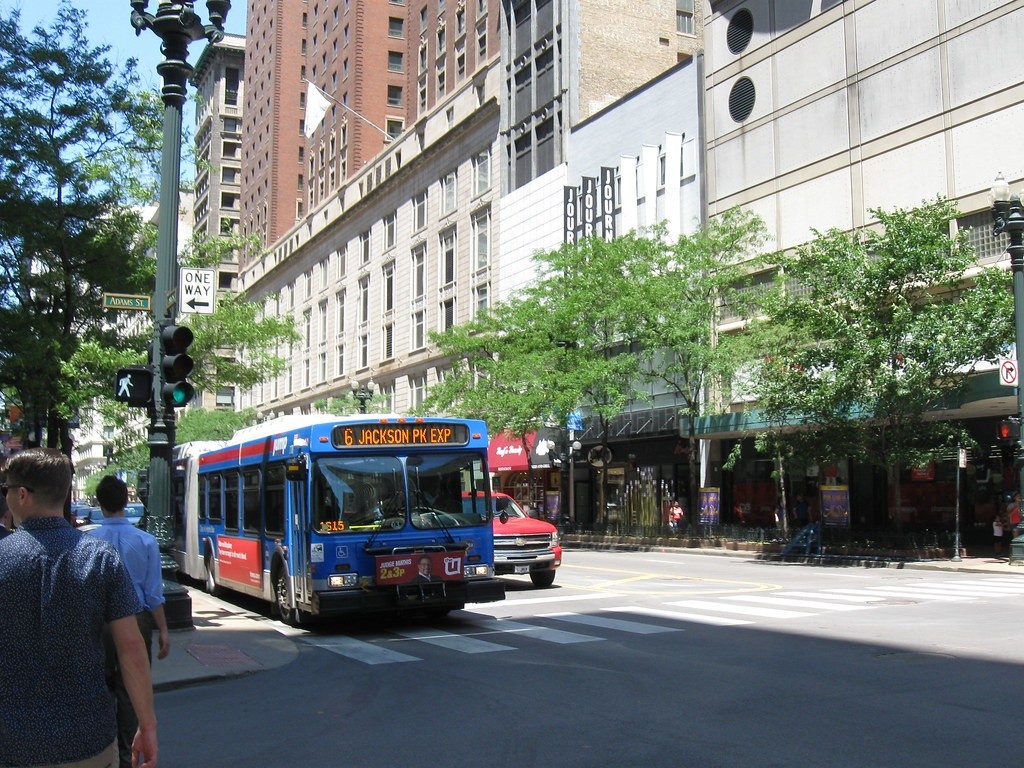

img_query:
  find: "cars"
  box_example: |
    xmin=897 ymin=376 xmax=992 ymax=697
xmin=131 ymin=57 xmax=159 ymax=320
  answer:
xmin=462 ymin=488 xmax=563 ymax=587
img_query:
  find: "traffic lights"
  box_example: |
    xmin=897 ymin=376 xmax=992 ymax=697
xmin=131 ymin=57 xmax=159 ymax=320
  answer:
xmin=115 ymin=367 xmax=154 ymax=404
xmin=158 ymin=318 xmax=195 ymax=409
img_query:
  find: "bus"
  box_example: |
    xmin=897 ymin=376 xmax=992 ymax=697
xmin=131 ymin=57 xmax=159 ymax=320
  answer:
xmin=171 ymin=410 xmax=511 ymax=626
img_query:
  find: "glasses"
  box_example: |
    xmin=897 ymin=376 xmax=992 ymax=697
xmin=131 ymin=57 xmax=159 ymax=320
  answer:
xmin=0 ymin=481 xmax=34 ymax=500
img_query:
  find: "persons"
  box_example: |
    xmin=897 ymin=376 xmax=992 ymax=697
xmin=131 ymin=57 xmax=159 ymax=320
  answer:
xmin=1 ymin=484 xmax=16 ymax=537
xmin=1006 ymin=490 xmax=1022 ymax=540
xmin=992 ymin=516 xmax=1007 ymax=554
xmin=668 ymin=501 xmax=683 ymax=537
xmin=82 ymin=475 xmax=171 ymax=766
xmin=793 ymin=493 xmax=813 ymax=528
xmin=1 ymin=448 xmax=159 ymax=768
xmin=774 ymin=499 xmax=789 ymax=528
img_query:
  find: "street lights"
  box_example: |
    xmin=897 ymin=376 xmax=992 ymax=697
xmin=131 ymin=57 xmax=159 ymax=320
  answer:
xmin=127 ymin=0 xmax=233 ymax=626
xmin=352 ymin=379 xmax=374 ymax=414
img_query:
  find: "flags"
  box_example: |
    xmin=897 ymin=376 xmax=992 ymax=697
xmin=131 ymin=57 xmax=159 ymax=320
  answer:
xmin=304 ymin=82 xmax=336 ymax=139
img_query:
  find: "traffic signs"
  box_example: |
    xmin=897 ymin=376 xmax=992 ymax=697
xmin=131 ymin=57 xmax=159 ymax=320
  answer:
xmin=180 ymin=268 xmax=215 ymax=314
